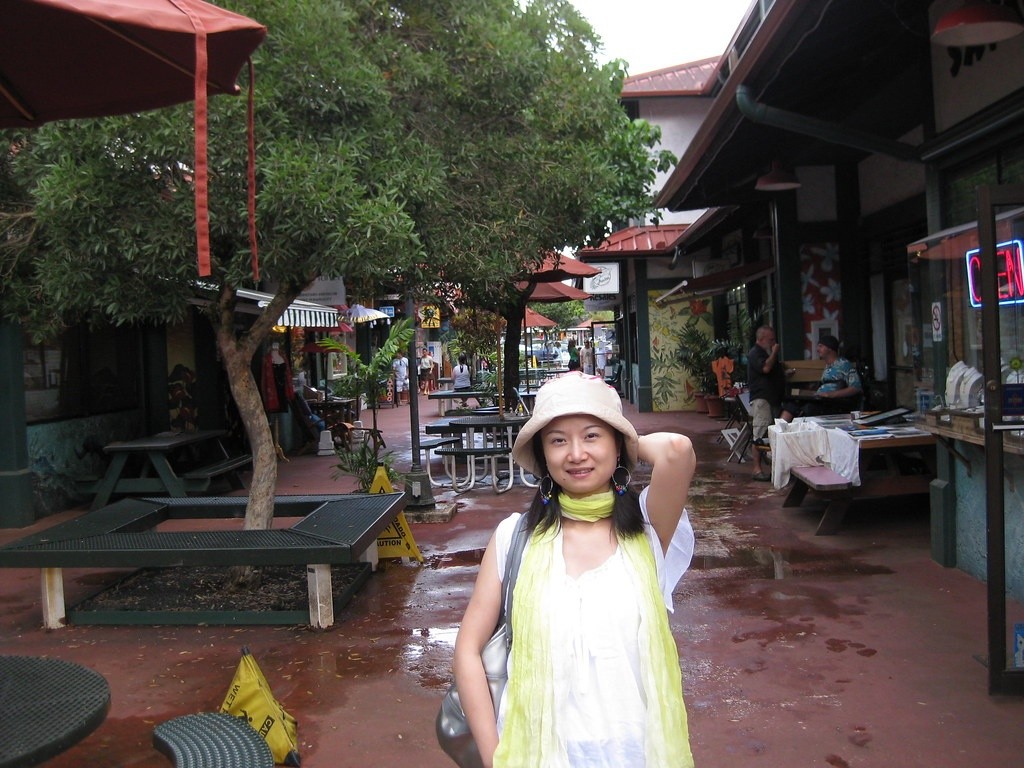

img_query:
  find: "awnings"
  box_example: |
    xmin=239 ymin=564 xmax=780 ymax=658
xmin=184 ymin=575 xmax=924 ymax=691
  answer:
xmin=656 ymin=261 xmax=775 ymax=306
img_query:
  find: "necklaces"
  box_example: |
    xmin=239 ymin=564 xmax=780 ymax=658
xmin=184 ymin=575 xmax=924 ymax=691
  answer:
xmin=1011 ymin=371 xmax=1023 ymax=376
xmin=949 ymin=366 xmax=976 ymax=393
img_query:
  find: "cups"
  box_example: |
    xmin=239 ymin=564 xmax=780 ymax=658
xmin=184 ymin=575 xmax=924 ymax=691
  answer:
xmin=851 ymin=411 xmax=860 ymax=419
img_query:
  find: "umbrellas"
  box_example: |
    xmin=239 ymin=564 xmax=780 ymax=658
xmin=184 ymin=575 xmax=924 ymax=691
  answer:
xmin=0 ymin=0 xmax=259 ymax=285
xmin=301 ymin=302 xmax=390 ymax=429
xmin=518 ymin=248 xmax=607 ymax=393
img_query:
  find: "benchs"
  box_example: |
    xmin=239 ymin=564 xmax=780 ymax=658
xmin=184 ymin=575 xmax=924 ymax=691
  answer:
xmin=78 ymin=453 xmax=256 ymax=510
xmin=1 ymin=488 xmax=405 ymax=629
xmin=765 ymin=450 xmax=936 ymax=535
xmin=153 ymin=714 xmax=276 ymax=767
xmin=421 ymin=370 xmax=568 ymax=493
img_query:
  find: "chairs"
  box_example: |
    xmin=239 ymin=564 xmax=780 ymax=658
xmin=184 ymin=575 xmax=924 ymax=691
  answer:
xmin=604 ymin=363 xmax=621 ymax=384
xmin=715 ymin=394 xmax=757 ymax=464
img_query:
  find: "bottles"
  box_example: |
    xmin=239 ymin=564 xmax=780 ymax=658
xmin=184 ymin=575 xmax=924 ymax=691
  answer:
xmin=509 ymin=400 xmax=513 ymax=417
xmin=519 ymin=403 xmax=523 ymax=413
xmin=515 ymin=400 xmax=520 ymax=416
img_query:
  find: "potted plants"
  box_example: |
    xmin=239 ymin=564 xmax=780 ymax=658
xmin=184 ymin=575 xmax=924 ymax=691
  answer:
xmin=678 ymin=309 xmax=770 ymax=419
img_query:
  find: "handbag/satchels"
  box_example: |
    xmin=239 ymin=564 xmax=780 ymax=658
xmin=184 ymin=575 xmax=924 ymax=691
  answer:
xmin=435 ymin=511 xmax=530 ymax=768
xmin=219 ymin=646 xmax=300 ymax=768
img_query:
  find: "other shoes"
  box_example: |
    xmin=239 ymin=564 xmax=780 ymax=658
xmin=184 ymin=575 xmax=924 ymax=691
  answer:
xmin=750 ymin=470 xmax=765 ymax=482
xmin=751 ymin=437 xmax=772 ymax=450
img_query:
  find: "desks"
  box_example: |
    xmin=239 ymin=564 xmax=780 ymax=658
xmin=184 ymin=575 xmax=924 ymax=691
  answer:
xmin=451 ymin=416 xmax=532 ymax=491
xmin=794 ymin=415 xmax=938 ymax=535
xmin=0 ymin=656 xmax=112 ymax=768
xmin=90 ymin=429 xmax=227 ymax=514
xmin=437 ymin=377 xmax=453 ymax=383
xmin=306 ymin=399 xmax=356 ymax=442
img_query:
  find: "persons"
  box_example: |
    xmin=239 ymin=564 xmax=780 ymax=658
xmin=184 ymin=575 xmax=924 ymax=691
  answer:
xmin=454 ymin=371 xmax=696 ymax=768
xmin=417 ymin=348 xmax=435 ymax=394
xmin=580 ymin=339 xmax=593 ymax=375
xmin=261 ymin=348 xmax=294 ymax=413
xmin=392 ymin=350 xmax=410 ymax=406
xmin=597 ymin=348 xmax=606 ymax=378
xmin=451 ymin=356 xmax=472 ymax=407
xmin=747 ymin=327 xmax=794 ymax=481
xmin=568 ymin=340 xmax=580 ymax=371
xmin=780 ymin=336 xmax=862 ymax=422
xmin=551 ymin=342 xmax=563 ymax=367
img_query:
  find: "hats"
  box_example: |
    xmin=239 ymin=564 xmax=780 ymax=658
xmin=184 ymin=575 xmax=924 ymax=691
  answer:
xmin=512 ymin=370 xmax=639 ymax=478
xmin=818 ymin=335 xmax=840 ymax=353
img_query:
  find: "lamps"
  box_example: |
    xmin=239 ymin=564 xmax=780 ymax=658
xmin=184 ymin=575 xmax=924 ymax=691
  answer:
xmin=755 ymin=151 xmax=802 ymax=191
xmin=929 ymin=0 xmax=1024 ymax=46
